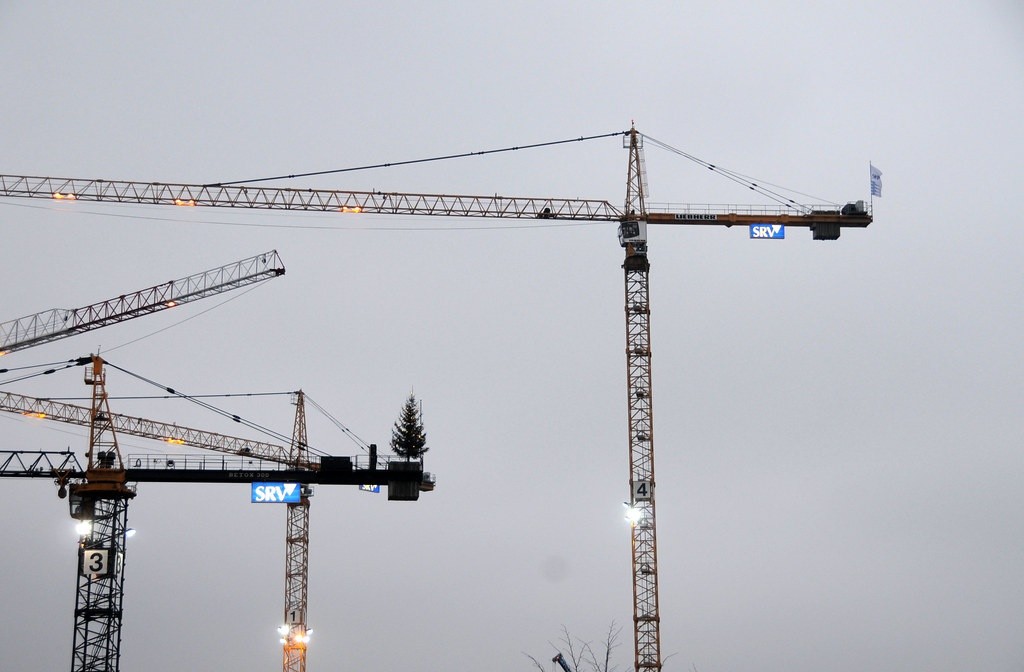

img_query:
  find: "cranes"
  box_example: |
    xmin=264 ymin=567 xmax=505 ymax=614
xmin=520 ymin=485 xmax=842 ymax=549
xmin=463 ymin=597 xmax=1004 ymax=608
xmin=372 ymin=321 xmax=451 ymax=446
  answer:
xmin=0 ymin=116 xmax=882 ymax=671
xmin=0 ymin=246 xmax=436 ymax=672
